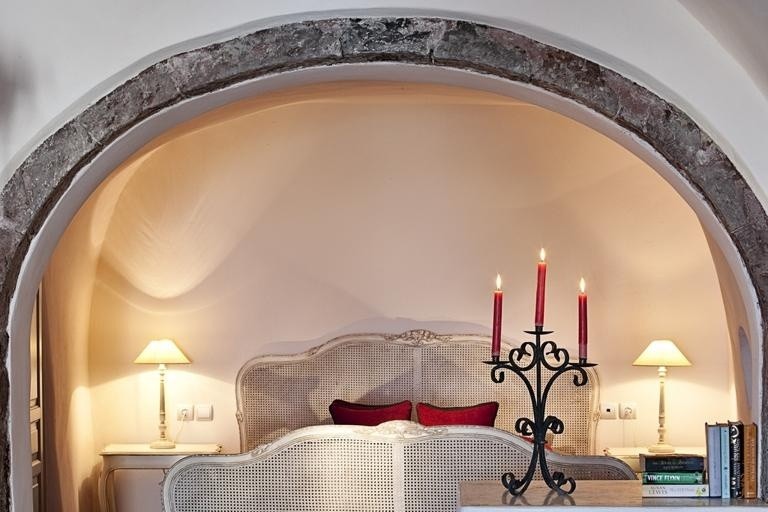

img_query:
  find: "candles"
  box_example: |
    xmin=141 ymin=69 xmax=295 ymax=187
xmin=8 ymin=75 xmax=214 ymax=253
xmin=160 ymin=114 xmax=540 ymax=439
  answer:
xmin=491 ymin=246 xmax=589 ymax=359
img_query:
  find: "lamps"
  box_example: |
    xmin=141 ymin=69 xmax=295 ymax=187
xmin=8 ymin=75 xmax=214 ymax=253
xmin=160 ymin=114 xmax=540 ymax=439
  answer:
xmin=134 ymin=337 xmax=191 ymax=447
xmin=631 ymin=338 xmax=693 ymax=455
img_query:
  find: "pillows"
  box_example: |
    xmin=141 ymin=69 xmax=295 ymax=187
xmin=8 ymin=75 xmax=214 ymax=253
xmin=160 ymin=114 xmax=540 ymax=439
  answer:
xmin=327 ymin=397 xmax=501 ymax=435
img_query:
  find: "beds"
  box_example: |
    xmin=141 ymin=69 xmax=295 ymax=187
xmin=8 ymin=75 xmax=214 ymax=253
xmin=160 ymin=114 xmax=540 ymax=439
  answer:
xmin=164 ymin=328 xmax=638 ymax=510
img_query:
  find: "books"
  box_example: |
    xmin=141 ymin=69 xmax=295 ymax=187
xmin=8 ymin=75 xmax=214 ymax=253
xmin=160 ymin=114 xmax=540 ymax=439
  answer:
xmin=716 ymin=421 xmax=731 ymax=499
xmin=639 ymin=453 xmax=704 ymax=473
xmin=728 ymin=420 xmax=744 ymax=499
xmin=705 ymin=421 xmax=721 ymax=498
xmin=743 ymin=422 xmax=757 ymax=499
xmin=641 ymin=483 xmax=710 ymax=498
xmin=641 ymin=472 xmax=705 ymax=484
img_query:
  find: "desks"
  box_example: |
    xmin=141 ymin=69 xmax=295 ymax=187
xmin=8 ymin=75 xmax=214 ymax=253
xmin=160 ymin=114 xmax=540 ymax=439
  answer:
xmin=96 ymin=442 xmax=222 ymax=512
xmin=455 ymin=478 xmax=767 ymax=511
